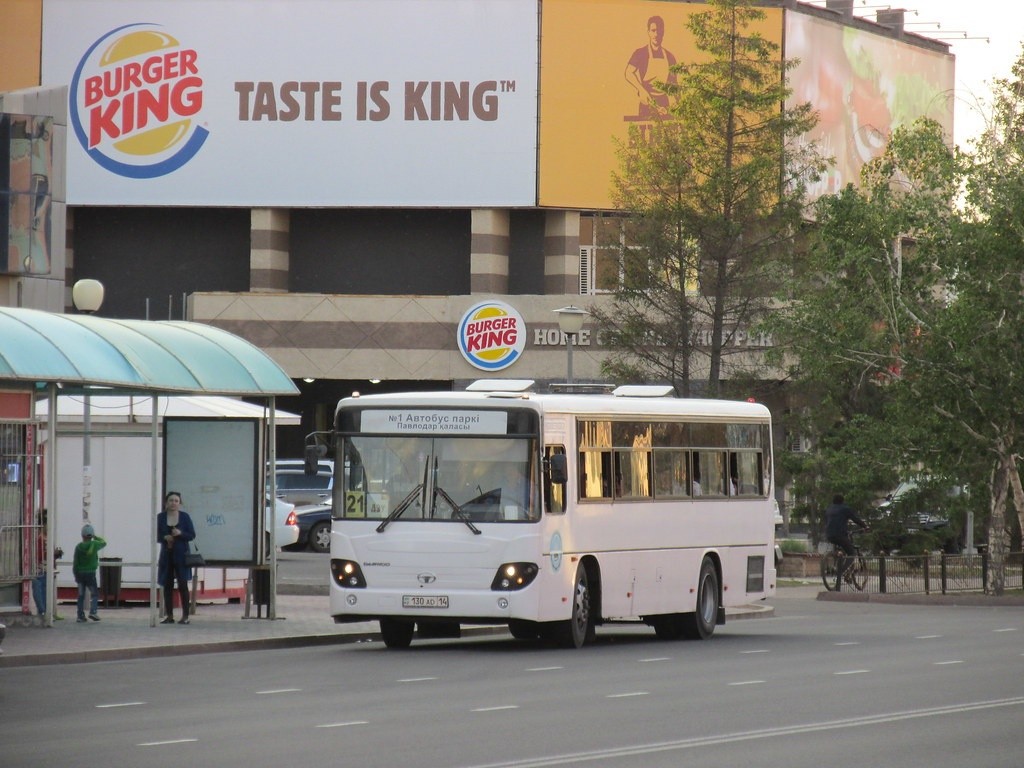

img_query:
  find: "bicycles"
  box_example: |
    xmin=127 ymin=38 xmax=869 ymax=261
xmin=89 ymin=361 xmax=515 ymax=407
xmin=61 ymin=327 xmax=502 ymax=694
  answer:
xmin=821 ymin=526 xmax=869 ymax=593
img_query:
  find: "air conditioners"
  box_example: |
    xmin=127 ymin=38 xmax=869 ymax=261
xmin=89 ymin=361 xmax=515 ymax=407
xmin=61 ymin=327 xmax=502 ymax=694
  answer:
xmin=579 ymin=248 xmax=590 ymax=295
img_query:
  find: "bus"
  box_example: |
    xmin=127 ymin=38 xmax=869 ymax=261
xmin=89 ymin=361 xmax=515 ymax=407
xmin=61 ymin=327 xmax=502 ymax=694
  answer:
xmin=304 ymin=378 xmax=776 ymax=647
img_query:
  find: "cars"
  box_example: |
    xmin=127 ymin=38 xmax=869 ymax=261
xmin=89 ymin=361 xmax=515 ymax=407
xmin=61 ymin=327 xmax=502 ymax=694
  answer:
xmin=265 ymin=492 xmax=332 ymax=553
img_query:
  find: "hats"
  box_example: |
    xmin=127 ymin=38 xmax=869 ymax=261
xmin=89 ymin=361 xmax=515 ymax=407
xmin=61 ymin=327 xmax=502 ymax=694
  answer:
xmin=81 ymin=524 xmax=94 ymax=538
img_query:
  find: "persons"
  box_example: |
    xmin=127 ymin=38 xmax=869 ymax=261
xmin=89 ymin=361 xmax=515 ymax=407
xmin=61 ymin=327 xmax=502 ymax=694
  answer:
xmin=388 ymin=455 xmax=415 ymax=493
xmin=157 ymin=493 xmax=195 ymax=624
xmin=604 ymin=465 xmax=736 ymax=498
xmin=490 ymin=466 xmax=538 ymax=511
xmin=826 ymin=495 xmax=870 ymax=581
xmin=73 ymin=524 xmax=106 ymax=622
xmin=34 ymin=509 xmax=65 ymax=621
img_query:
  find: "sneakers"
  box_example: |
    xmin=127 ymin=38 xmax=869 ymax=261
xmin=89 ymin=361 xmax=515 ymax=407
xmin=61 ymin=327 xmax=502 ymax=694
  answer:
xmin=88 ymin=613 xmax=100 ymax=621
xmin=77 ymin=617 xmax=88 ymax=622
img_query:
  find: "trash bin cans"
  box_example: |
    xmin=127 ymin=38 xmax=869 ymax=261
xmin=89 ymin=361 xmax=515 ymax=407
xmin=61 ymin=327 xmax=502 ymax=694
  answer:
xmin=99 ymin=557 xmax=123 ymax=608
xmin=251 ymin=563 xmax=280 ymax=618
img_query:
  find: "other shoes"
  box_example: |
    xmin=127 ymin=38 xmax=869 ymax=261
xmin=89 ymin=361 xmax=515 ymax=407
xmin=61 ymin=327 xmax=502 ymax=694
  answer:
xmin=160 ymin=617 xmax=175 ymax=624
xmin=44 ymin=614 xmax=64 ymax=622
xmin=178 ymin=619 xmax=189 ymax=625
xmin=845 ymin=577 xmax=852 ymax=584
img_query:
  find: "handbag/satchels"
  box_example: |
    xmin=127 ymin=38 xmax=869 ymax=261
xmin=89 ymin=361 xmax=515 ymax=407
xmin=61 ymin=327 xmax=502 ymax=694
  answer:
xmin=184 ymin=536 xmax=206 ymax=568
